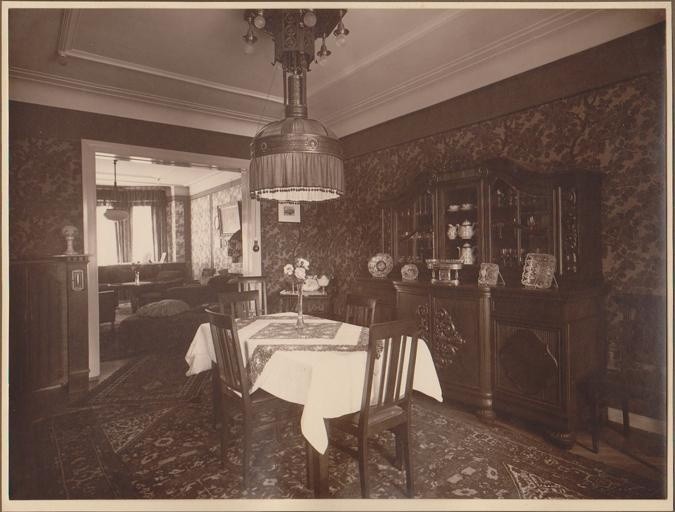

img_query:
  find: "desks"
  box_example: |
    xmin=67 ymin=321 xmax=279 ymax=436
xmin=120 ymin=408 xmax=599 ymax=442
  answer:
xmin=182 ymin=310 xmax=446 ymax=496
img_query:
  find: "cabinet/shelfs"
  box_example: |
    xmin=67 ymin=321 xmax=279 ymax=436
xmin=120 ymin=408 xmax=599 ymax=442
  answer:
xmin=356 ymin=157 xmax=608 ymax=449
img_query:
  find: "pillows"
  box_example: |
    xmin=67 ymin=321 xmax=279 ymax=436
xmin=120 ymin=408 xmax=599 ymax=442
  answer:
xmin=156 ymin=270 xmax=183 ymax=281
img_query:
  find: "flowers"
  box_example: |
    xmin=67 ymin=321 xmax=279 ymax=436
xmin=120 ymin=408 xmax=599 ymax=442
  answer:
xmin=284 ymin=257 xmax=310 ymax=279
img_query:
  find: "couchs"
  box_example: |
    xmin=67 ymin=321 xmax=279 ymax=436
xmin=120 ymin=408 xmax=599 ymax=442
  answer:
xmin=98 ymin=263 xmax=194 ymax=301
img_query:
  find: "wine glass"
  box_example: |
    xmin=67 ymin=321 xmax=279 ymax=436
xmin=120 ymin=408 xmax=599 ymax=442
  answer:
xmin=499 ymin=247 xmax=525 ymax=266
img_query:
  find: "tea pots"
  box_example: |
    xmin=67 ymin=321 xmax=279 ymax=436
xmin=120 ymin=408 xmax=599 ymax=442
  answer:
xmin=446 ymin=218 xmax=479 ymax=264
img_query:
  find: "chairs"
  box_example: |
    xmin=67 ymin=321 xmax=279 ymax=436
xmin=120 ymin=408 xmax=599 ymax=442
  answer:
xmin=216 ymin=288 xmax=265 ymax=322
xmin=341 ymin=292 xmax=382 ymax=328
xmin=304 ymin=315 xmax=421 ymax=500
xmin=204 ymin=306 xmax=302 ymax=489
xmin=584 ymin=293 xmax=667 ymax=454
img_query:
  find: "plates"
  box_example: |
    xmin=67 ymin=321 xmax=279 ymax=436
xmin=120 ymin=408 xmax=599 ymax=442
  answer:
xmin=365 ymin=252 xmax=419 ymax=280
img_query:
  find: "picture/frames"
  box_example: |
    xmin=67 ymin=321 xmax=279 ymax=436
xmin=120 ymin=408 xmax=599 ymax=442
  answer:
xmin=216 ymin=201 xmax=241 ymax=236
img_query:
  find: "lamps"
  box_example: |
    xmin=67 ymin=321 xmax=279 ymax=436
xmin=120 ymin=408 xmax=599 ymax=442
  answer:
xmin=104 ymin=160 xmax=130 ymax=222
xmin=241 ymin=8 xmax=351 ymax=203
